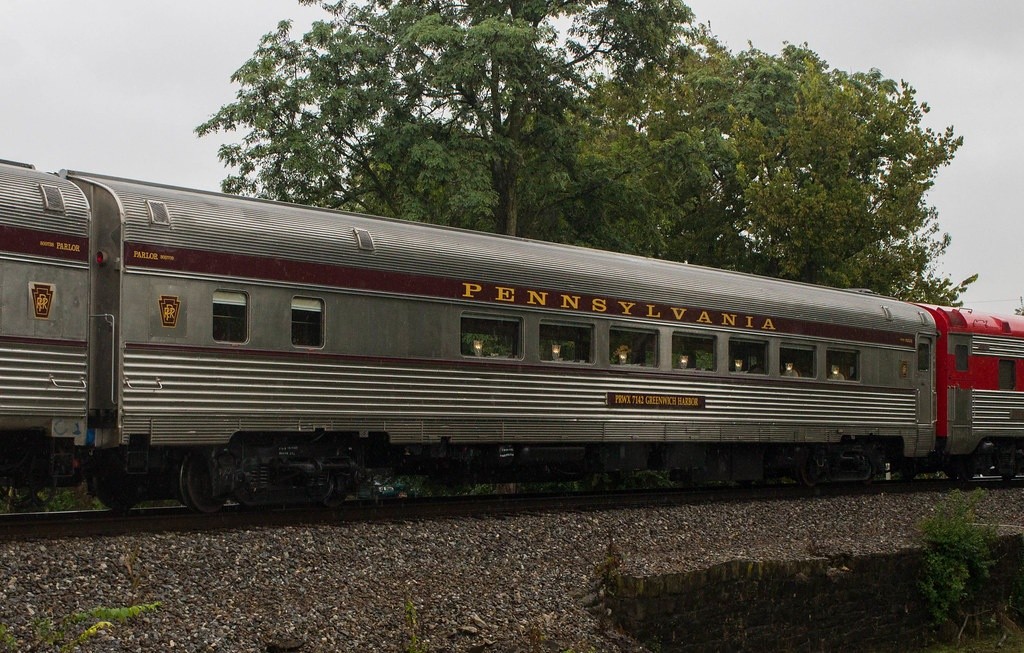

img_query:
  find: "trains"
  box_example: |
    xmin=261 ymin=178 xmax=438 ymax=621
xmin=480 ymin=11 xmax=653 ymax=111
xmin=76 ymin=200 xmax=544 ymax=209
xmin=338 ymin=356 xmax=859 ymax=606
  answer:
xmin=0 ymin=158 xmax=1024 ymax=513
